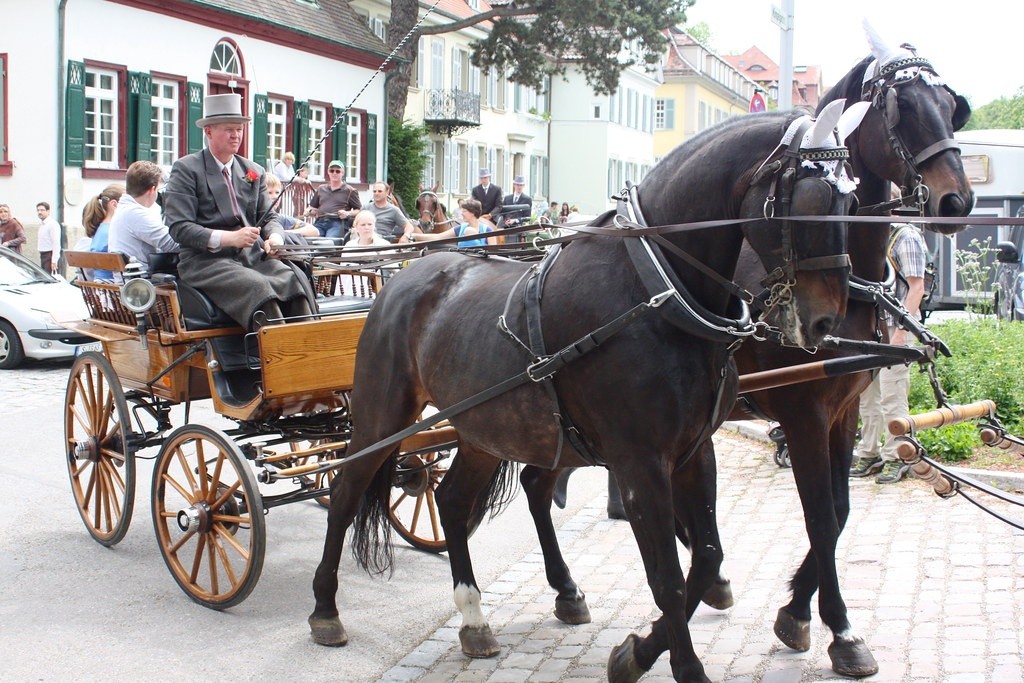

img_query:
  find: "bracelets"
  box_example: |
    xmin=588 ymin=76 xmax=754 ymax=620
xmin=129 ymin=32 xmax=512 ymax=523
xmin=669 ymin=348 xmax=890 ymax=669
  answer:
xmin=489 ymin=213 xmax=492 ymax=218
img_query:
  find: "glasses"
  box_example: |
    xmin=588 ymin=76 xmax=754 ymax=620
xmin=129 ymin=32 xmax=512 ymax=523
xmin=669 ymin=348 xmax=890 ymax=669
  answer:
xmin=328 ymin=170 xmax=343 ymax=173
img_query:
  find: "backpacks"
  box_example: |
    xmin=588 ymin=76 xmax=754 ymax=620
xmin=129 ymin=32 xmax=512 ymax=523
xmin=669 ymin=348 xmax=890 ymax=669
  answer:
xmin=887 ymin=225 xmax=936 ymax=308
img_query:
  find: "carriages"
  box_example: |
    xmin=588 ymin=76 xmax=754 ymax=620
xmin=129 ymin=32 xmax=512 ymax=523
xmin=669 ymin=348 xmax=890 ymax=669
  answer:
xmin=61 ymin=22 xmax=977 ymax=683
xmin=414 ymin=180 xmax=595 ymax=262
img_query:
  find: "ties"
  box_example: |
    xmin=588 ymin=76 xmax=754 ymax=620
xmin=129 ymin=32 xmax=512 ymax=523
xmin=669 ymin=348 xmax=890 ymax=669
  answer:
xmin=222 ymin=167 xmax=241 ymax=215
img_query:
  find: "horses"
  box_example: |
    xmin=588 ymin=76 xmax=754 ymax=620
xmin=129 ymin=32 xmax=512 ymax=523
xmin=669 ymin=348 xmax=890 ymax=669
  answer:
xmin=415 ymin=180 xmax=452 ymax=233
xmin=308 ymin=98 xmax=859 ymax=683
xmin=518 ymin=42 xmax=980 ymax=679
xmin=369 ymin=181 xmax=423 ymax=251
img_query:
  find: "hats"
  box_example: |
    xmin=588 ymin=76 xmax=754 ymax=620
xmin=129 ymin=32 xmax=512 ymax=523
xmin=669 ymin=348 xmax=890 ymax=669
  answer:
xmin=195 ymin=94 xmax=251 ymax=128
xmin=327 ymin=160 xmax=344 ymax=169
xmin=512 ymin=176 xmax=526 ymax=184
xmin=478 ymin=169 xmax=491 ymax=178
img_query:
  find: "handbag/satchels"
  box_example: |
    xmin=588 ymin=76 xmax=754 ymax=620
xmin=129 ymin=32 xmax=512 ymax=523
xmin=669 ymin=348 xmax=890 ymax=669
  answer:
xmin=50 ymin=269 xmax=66 ymax=281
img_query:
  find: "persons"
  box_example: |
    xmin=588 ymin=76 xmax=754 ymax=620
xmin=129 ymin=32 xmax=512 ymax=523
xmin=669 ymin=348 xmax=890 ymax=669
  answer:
xmin=74 ymin=185 xmax=123 ymax=312
xmin=359 ymin=182 xmax=413 ymax=252
xmin=37 ymin=202 xmax=61 ymax=276
xmin=501 ymin=175 xmax=532 ymax=227
xmin=273 ymin=152 xmax=312 ymax=219
xmin=107 ymin=160 xmax=180 ymax=285
xmin=0 ymin=204 xmax=27 ymax=264
xmin=163 ymin=94 xmax=320 ymax=336
xmin=471 ymin=167 xmax=503 ymax=226
xmin=304 ymin=160 xmax=363 ymax=250
xmin=411 ymin=200 xmax=497 ymax=256
xmin=554 ymin=467 xmax=629 ymax=522
xmin=848 ymin=181 xmax=926 ymax=485
xmin=334 ymin=210 xmax=400 ymax=300
xmin=541 ymin=201 xmax=578 ymax=224
xmin=265 ymin=172 xmax=320 ymax=237
xmin=451 ymin=199 xmax=467 ymax=226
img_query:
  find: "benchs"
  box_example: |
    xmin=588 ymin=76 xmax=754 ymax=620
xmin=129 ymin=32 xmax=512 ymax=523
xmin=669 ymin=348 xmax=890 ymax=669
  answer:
xmin=64 ymin=248 xmax=376 ymax=340
xmin=503 ymin=204 xmax=530 ymax=228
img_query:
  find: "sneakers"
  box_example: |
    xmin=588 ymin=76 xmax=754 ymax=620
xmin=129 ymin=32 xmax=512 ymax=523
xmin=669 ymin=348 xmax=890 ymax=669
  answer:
xmin=875 ymin=459 xmax=909 ymax=483
xmin=848 ymin=456 xmax=883 ymax=476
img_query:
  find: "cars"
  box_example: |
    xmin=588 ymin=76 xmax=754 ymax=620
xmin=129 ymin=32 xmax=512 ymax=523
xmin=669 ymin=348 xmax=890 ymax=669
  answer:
xmin=0 ymin=243 xmax=106 ymax=370
